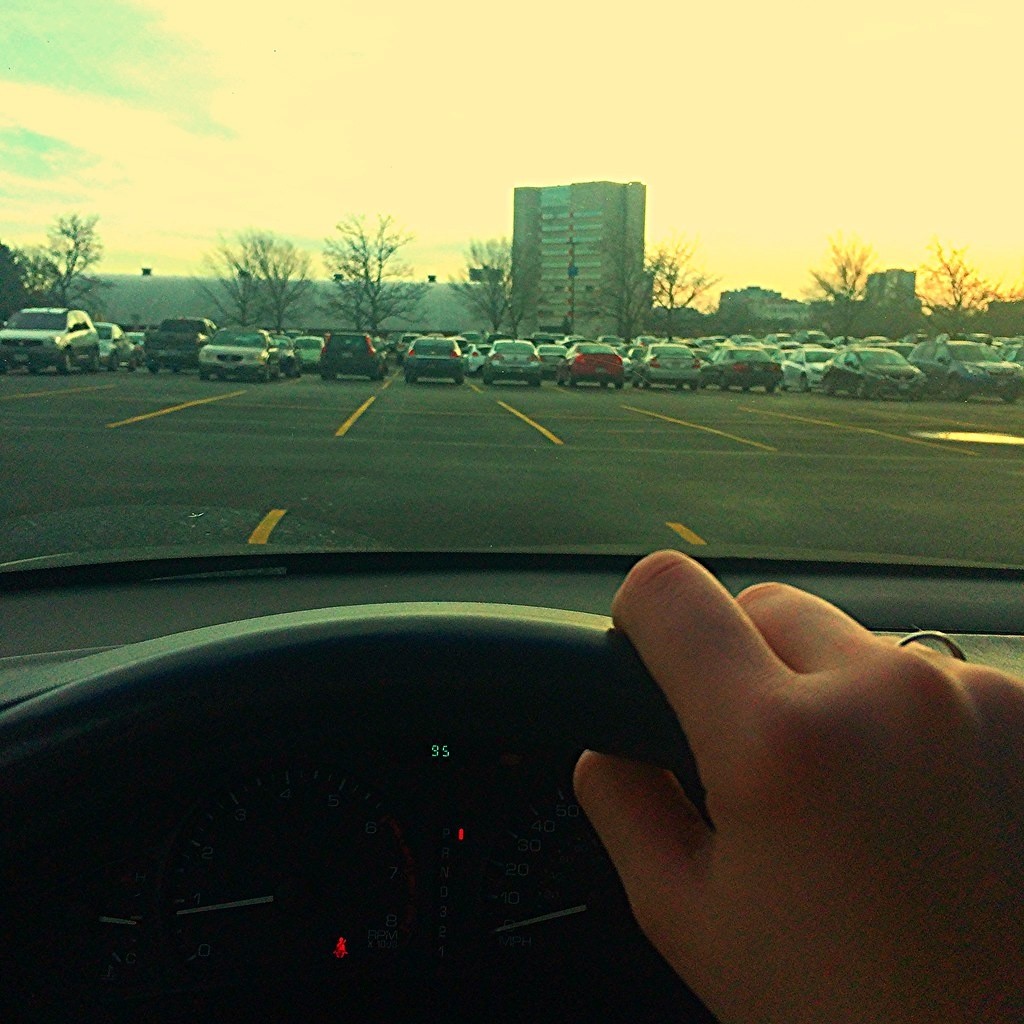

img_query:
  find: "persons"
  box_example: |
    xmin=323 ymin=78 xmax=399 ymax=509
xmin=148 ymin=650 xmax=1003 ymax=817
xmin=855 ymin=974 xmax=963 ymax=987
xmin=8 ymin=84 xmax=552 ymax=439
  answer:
xmin=574 ymin=551 xmax=1023 ymax=1024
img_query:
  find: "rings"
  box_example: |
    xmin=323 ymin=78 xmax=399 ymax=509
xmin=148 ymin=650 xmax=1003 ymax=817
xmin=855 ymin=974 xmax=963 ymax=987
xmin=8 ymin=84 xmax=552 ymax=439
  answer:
xmin=900 ymin=631 xmax=968 ymax=663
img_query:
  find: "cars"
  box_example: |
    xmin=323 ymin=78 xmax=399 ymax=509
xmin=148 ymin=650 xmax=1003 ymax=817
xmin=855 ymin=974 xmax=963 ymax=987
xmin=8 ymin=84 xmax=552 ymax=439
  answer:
xmin=0 ymin=307 xmax=99 ymax=375
xmin=633 ymin=344 xmax=701 ymax=391
xmin=482 ymin=338 xmax=542 ymax=386
xmin=702 ymin=346 xmax=781 ymax=393
xmin=95 ymin=317 xmax=323 ymax=383
xmin=823 ymin=348 xmax=923 ymax=402
xmin=397 ymin=332 xmax=1024 ymax=403
xmin=319 ymin=333 xmax=387 ymax=382
xmin=554 ymin=343 xmax=625 ymax=390
xmin=403 ymin=337 xmax=466 ymax=384
